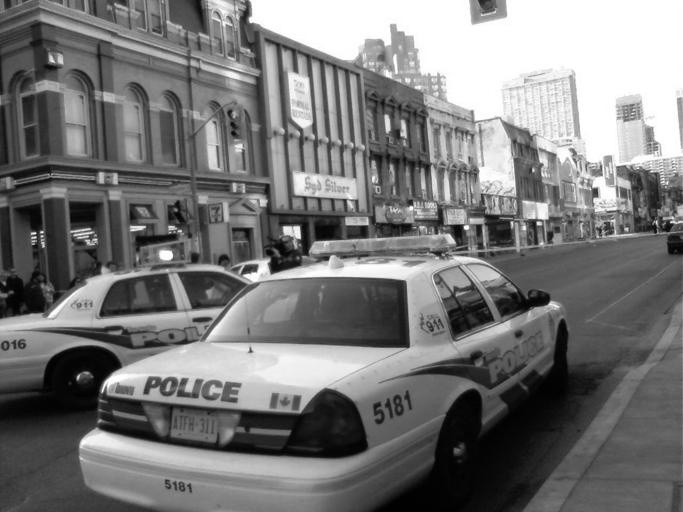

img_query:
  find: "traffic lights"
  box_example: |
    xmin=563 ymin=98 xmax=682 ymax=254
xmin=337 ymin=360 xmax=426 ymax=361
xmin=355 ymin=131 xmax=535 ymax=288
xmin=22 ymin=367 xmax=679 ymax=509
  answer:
xmin=223 ymin=103 xmax=248 ymax=148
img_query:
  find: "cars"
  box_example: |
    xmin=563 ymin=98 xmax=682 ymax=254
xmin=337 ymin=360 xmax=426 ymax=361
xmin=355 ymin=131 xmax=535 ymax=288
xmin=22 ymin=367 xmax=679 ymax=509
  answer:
xmin=666 ymin=221 xmax=683 ymax=255
xmin=0 ymin=259 xmax=256 ymax=410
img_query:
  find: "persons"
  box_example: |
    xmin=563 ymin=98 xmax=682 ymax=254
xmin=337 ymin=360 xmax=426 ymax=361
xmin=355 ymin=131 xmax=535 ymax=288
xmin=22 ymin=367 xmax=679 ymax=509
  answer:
xmin=105 ymin=260 xmax=117 ymax=272
xmin=37 ymin=272 xmax=55 ymax=310
xmin=183 ymin=252 xmax=214 ymax=299
xmin=4 ymin=268 xmax=24 ymax=315
xmin=23 ymin=271 xmax=44 ymax=313
xmin=0 ymin=270 xmax=14 ymax=318
xmin=268 ymin=235 xmax=301 ymax=275
xmin=94 ymin=262 xmax=102 ymax=275
xmin=217 ymin=254 xmax=229 ymax=268
